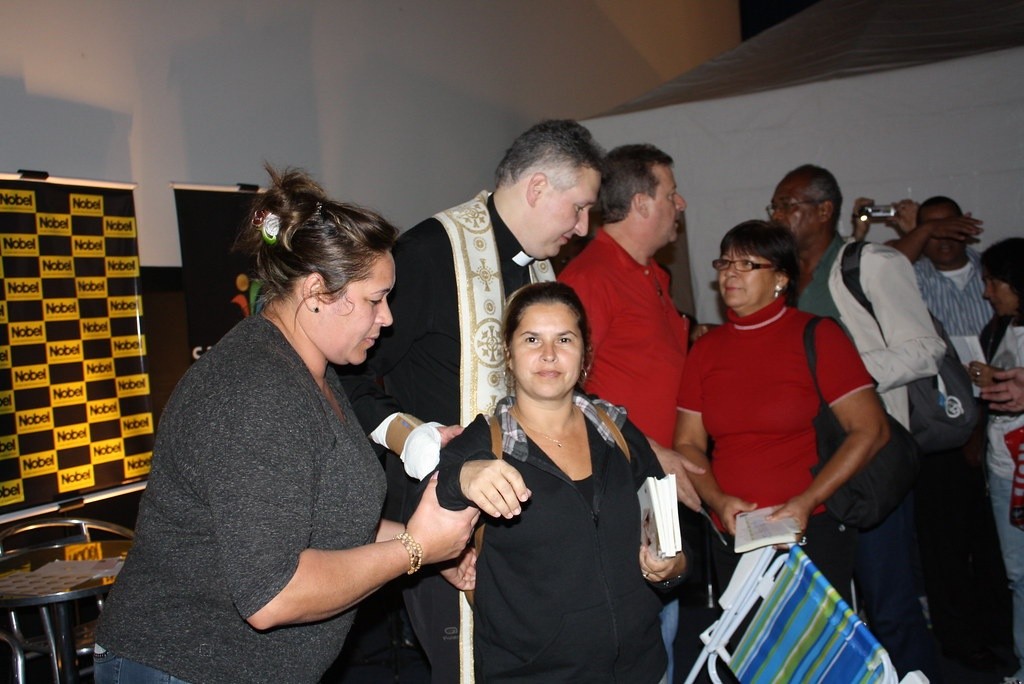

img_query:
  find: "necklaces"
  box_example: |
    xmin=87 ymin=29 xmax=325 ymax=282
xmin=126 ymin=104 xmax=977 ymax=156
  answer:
xmin=512 ymin=406 xmax=576 ymax=448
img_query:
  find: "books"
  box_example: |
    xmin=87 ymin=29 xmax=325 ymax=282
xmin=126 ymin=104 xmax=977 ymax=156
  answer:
xmin=734 ymin=504 xmax=796 ymax=553
xmin=636 ymin=473 xmax=682 ymax=561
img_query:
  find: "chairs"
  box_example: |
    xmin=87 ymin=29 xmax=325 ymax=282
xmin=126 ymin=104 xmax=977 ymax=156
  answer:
xmin=0 ymin=517 xmax=137 ymax=684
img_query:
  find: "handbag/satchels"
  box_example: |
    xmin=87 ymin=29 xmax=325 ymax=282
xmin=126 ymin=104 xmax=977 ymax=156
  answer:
xmin=804 ymin=314 xmax=923 ymax=529
xmin=841 ymin=239 xmax=980 ymax=452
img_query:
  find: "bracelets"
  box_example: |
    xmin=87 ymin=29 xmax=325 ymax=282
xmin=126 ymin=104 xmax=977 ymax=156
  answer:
xmin=394 ymin=533 xmax=422 ymax=576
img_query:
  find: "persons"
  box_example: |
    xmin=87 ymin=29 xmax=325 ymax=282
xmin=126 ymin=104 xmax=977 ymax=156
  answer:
xmin=672 ymin=221 xmax=889 ymax=684
xmin=765 ymin=163 xmax=1024 ymax=684
xmin=416 ymin=280 xmax=709 ymax=684
xmin=554 ymin=143 xmax=690 ymax=684
xmin=93 ymin=166 xmax=481 ymax=684
xmin=327 ymin=117 xmax=604 ymax=684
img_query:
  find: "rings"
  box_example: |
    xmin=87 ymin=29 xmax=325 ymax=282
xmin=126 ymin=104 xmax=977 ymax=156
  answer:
xmin=976 ymin=371 xmax=980 ymax=377
xmin=644 ymin=573 xmax=649 ymax=577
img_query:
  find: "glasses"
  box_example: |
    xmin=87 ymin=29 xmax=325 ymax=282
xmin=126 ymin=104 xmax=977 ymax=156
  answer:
xmin=766 ymin=200 xmax=825 ymax=218
xmin=713 ymin=260 xmax=776 ymax=271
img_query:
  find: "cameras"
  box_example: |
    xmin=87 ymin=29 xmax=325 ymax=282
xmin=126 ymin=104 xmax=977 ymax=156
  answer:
xmin=859 ymin=205 xmax=897 ymax=222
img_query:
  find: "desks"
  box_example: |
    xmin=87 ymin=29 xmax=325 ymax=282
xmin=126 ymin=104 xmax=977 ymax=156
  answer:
xmin=0 ymin=539 xmax=136 ymax=684
